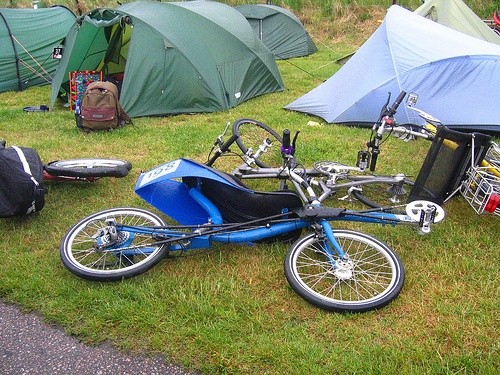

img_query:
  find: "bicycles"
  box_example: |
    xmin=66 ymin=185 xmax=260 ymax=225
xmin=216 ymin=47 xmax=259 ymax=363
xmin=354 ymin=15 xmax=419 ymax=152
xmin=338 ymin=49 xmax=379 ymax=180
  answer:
xmin=361 ymin=90 xmax=500 ymax=217
xmin=60 ymin=129 xmax=405 ymax=314
xmin=202 ymin=119 xmax=444 ymax=216
xmin=44 ymin=156 xmax=132 ymax=183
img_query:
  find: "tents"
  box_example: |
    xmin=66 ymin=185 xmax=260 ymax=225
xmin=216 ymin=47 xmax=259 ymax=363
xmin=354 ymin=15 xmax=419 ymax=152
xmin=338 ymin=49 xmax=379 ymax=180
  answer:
xmin=235 ymin=4 xmax=318 ymax=59
xmin=282 ymin=4 xmax=500 ymax=132
xmin=0 ymin=0 xmax=77 ymax=90
xmin=334 ymin=0 xmax=500 ymax=62
xmin=49 ymin=0 xmax=285 ymax=118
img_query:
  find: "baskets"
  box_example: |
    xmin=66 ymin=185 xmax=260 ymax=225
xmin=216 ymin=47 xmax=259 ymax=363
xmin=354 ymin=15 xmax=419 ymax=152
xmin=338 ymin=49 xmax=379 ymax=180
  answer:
xmin=460 ymin=166 xmax=500 ymax=216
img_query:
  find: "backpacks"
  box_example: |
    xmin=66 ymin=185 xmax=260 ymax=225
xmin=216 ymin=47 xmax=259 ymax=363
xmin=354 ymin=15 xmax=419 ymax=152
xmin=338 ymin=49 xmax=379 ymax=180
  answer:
xmin=75 ymin=81 xmax=133 ymax=132
